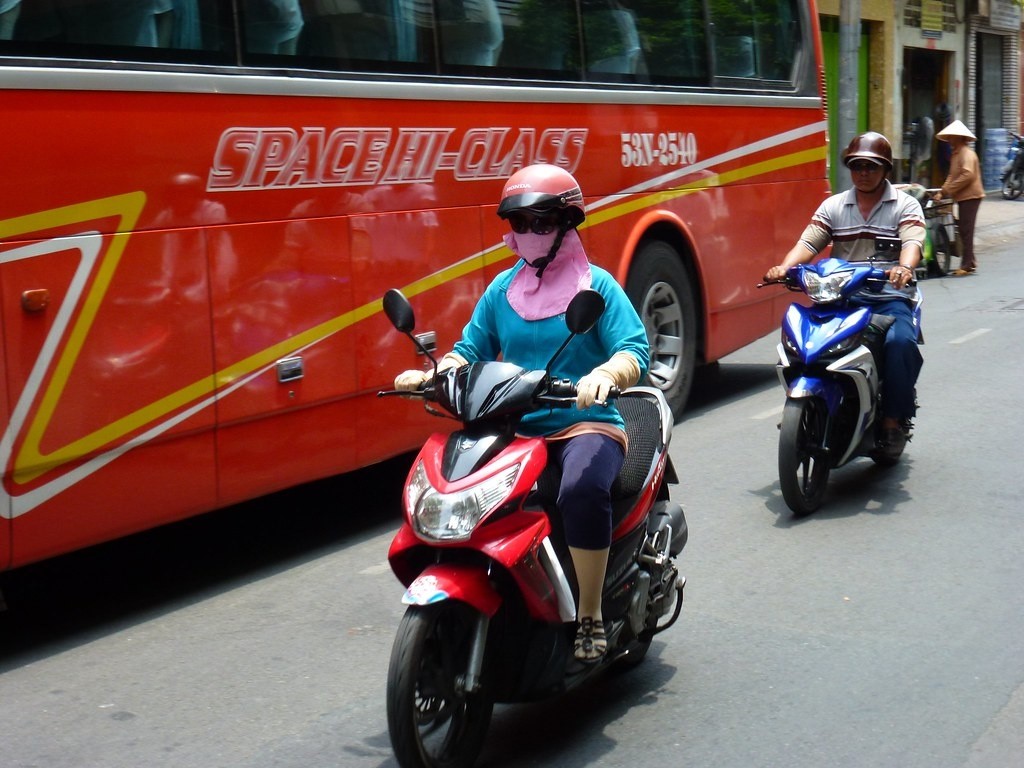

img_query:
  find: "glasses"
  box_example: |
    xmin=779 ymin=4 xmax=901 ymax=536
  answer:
xmin=854 ymin=162 xmax=878 ymax=171
xmin=508 ymin=215 xmax=558 ymax=235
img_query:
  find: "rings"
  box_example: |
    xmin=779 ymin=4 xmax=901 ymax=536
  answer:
xmin=896 ymin=270 xmax=902 ymax=274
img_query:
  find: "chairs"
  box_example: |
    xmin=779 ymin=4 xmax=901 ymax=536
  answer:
xmin=238 ymin=0 xmax=758 ymax=78
xmin=110 ymin=0 xmax=204 ymax=50
xmin=0 ymin=0 xmax=22 ymax=40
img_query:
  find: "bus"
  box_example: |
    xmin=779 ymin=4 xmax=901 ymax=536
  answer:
xmin=0 ymin=0 xmax=830 ymax=573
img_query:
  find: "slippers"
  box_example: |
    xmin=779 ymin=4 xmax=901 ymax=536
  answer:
xmin=953 ymin=267 xmax=975 ymax=276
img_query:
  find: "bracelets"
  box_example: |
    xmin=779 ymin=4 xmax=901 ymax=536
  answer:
xmin=904 ymin=264 xmax=911 ymax=268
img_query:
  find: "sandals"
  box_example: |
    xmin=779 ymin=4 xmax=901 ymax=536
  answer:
xmin=572 ymin=616 xmax=609 ymax=664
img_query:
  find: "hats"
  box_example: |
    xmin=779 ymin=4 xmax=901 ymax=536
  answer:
xmin=935 ymin=118 xmax=977 ymax=143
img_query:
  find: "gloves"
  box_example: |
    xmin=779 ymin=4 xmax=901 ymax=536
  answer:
xmin=394 ymin=350 xmax=469 ymax=396
xmin=577 ymin=349 xmax=642 ymax=408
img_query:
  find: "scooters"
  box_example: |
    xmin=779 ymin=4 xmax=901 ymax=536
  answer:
xmin=376 ymin=288 xmax=688 ymax=768
xmin=757 ymin=256 xmax=926 ymax=516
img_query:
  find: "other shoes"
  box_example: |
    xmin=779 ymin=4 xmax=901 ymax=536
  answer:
xmin=880 ymin=414 xmax=900 ymax=445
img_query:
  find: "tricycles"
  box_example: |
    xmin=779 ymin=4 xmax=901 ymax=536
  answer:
xmin=895 ymin=184 xmax=962 ymax=277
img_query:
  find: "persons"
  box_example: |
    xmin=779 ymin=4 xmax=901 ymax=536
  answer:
xmin=394 ymin=165 xmax=650 ymax=664
xmin=767 ymin=131 xmax=927 ymax=461
xmin=933 ymin=120 xmax=986 ymax=276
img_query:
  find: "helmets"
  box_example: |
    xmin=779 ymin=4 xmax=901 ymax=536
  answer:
xmin=497 ymin=164 xmax=586 ymax=229
xmin=842 ymin=130 xmax=894 ymax=169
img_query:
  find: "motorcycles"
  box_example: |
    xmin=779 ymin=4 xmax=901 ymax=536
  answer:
xmin=1000 ymin=130 xmax=1024 ymax=198
